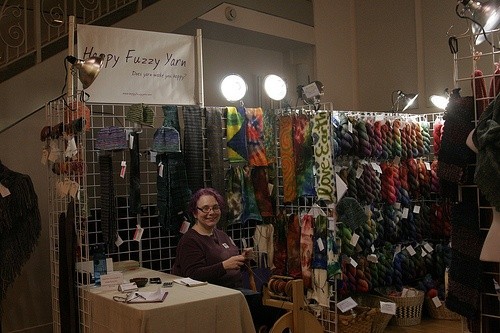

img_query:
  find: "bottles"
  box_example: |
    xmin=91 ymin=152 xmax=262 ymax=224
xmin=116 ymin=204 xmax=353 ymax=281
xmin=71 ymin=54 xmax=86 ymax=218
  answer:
xmin=93 ymin=247 xmax=108 ymax=284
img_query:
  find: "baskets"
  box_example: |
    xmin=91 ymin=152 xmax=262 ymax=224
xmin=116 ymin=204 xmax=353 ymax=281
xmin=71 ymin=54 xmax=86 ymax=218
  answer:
xmin=426 ymin=295 xmax=465 ymax=320
xmin=383 ymin=290 xmax=424 ymax=326
xmin=354 ymin=294 xmax=396 ymax=333
xmin=320 ymin=299 xmax=376 ymax=333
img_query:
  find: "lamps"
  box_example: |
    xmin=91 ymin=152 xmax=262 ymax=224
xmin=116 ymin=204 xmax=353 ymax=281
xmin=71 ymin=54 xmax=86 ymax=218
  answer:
xmin=296 ymin=81 xmax=325 ymax=105
xmin=394 ymin=90 xmax=419 ymax=113
xmin=66 ymin=55 xmax=105 ymax=90
xmin=430 ymin=88 xmax=450 ymax=111
xmin=263 ymin=71 xmax=288 ymax=100
xmin=457 ymin=0 xmax=500 ymax=46
xmin=218 ymin=73 xmax=249 ymax=102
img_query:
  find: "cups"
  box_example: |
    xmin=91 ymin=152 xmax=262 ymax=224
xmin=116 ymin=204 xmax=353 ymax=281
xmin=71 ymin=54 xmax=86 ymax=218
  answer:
xmin=243 ymin=247 xmax=253 ymax=256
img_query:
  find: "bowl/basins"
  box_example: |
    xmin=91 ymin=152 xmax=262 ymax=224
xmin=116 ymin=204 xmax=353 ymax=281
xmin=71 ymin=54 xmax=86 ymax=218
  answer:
xmin=130 ymin=278 xmax=148 ymax=287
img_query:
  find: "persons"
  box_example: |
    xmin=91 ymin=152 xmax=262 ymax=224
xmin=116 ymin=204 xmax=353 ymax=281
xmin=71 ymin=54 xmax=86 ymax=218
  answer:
xmin=172 ymin=188 xmax=255 ymax=291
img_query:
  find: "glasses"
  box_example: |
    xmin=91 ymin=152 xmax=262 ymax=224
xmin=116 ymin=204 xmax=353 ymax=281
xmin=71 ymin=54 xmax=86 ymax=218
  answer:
xmin=196 ymin=205 xmax=220 ymax=213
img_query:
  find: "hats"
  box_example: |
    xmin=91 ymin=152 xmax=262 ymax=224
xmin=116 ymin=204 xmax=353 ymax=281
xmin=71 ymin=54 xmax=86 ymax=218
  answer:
xmin=95 ymin=126 xmax=128 ymax=150
xmin=127 ymin=103 xmax=154 ymax=126
xmin=336 ymin=197 xmax=368 ymax=226
xmin=153 ymin=127 xmax=180 ymax=152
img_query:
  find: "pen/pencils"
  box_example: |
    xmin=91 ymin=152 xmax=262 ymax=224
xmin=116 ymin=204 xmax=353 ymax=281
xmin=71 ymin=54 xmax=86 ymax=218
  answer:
xmin=135 ymin=293 xmax=146 ymax=300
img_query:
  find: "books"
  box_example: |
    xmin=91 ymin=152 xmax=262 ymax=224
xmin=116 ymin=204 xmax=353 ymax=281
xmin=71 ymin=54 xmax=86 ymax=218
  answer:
xmin=174 ymin=277 xmax=208 ymax=287
xmin=128 ymin=288 xmax=168 ymax=303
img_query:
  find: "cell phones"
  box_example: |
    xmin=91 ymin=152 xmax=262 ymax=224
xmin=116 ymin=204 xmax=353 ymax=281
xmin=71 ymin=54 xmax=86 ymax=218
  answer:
xmin=150 ymin=278 xmax=161 ymax=284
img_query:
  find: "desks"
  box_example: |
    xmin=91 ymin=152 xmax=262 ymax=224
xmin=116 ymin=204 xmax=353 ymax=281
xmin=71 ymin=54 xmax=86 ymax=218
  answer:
xmin=384 ymin=317 xmax=462 ymax=333
xmin=78 ymin=269 xmax=256 ymax=333
